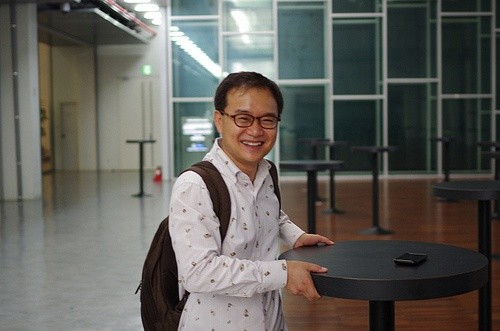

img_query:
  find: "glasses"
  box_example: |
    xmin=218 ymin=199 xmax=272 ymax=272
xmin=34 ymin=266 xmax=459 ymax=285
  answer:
xmin=221 ymin=109 xmax=282 ymax=129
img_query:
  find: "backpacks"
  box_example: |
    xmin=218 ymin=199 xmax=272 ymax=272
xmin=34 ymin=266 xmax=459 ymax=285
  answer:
xmin=134 ymin=160 xmax=282 ymax=331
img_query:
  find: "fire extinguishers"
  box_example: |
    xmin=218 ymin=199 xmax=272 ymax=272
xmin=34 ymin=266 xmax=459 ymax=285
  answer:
xmin=153 ymin=166 xmax=162 ymax=182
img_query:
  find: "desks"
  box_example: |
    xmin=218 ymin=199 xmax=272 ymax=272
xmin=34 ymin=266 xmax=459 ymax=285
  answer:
xmin=431 ymin=138 xmax=457 ymax=201
xmin=299 ymin=138 xmax=327 ymax=202
xmin=278 ymin=240 xmax=489 ymax=331
xmin=279 ymin=160 xmax=344 ymax=235
xmin=315 ymin=140 xmax=350 ymax=214
xmin=432 ymin=179 xmax=500 ymax=331
xmin=354 ymin=145 xmax=398 ymax=234
xmin=127 ymin=140 xmax=156 ymax=198
xmin=478 ymin=151 xmax=500 ymax=220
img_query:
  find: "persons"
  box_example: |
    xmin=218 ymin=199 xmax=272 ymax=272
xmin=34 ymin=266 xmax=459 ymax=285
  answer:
xmin=169 ymin=71 xmax=335 ymax=331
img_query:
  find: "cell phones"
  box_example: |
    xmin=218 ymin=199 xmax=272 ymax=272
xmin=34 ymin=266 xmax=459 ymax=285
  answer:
xmin=393 ymin=252 xmax=427 ymax=265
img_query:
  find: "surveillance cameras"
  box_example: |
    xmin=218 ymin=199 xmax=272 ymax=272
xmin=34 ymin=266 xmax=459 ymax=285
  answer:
xmin=59 ymin=3 xmax=71 ymax=15
